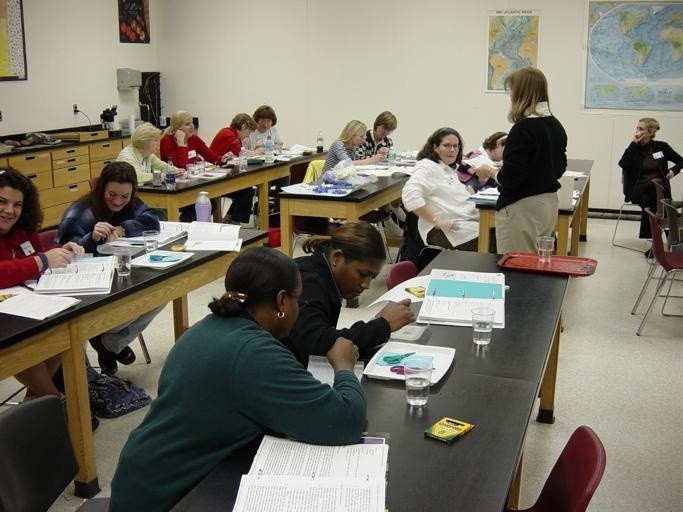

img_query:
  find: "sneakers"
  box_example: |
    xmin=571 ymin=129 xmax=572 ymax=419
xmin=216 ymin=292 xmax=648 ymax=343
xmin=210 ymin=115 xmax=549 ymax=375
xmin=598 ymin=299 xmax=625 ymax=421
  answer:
xmin=89 ymin=336 xmax=136 ymax=365
xmin=96 ymin=351 xmax=119 ymax=375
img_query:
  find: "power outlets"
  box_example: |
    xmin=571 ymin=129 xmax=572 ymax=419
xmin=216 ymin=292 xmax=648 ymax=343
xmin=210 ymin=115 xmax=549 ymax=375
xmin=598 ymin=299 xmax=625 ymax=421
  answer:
xmin=73 ymin=103 xmax=78 ymax=115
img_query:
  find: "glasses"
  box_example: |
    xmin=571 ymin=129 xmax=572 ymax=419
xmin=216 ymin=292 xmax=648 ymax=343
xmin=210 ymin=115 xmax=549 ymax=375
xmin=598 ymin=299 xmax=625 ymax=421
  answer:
xmin=285 ymin=292 xmax=310 ymax=309
xmin=439 ymin=142 xmax=462 ymax=150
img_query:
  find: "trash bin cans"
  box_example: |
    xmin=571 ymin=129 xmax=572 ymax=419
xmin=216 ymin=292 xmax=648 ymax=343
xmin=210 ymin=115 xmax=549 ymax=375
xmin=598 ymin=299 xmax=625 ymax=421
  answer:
xmin=252 ymin=198 xmax=281 ymax=248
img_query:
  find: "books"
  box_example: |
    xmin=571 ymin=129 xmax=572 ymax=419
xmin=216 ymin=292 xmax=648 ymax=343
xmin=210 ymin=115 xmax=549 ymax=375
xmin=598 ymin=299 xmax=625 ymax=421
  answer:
xmin=0 ymin=222 xmax=244 ymax=321
xmin=228 ymin=433 xmax=389 ymax=512
xmin=417 ymin=267 xmax=506 ymax=329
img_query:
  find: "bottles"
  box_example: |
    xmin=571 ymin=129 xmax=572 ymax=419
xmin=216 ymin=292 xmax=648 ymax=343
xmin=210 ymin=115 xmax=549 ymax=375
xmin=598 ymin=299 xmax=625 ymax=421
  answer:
xmin=194 ymin=192 xmax=211 ymax=222
xmin=387 ymin=147 xmax=396 ymax=169
xmin=238 ymin=147 xmax=247 ymax=171
xmin=164 ymin=156 xmax=175 ymax=190
xmin=316 ymin=130 xmax=324 ymax=153
xmin=264 ymin=136 xmax=274 ymax=164
xmin=573 ymin=177 xmax=580 ymax=199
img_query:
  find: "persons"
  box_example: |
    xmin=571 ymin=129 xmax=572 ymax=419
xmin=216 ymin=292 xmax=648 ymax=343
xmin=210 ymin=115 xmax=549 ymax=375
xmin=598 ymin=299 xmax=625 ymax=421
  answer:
xmin=617 ymin=118 xmax=683 ymax=259
xmin=0 ymin=165 xmax=104 ymax=436
xmin=458 ymin=132 xmax=510 ymax=193
xmin=270 ymin=220 xmax=415 ymax=358
xmin=354 ymin=110 xmax=408 ymax=237
xmin=240 ymin=105 xmax=289 ymax=214
xmin=157 ymin=110 xmax=234 ymax=223
xmin=52 ymin=160 xmax=172 ymax=375
xmin=103 ymin=244 xmax=367 ymax=511
xmin=207 ymin=113 xmax=265 ymax=225
xmin=319 ymin=120 xmax=385 ymax=180
xmin=475 ymin=66 xmax=568 ymax=257
xmin=112 ymin=121 xmax=186 ymax=220
xmin=399 ymin=126 xmax=497 ymax=257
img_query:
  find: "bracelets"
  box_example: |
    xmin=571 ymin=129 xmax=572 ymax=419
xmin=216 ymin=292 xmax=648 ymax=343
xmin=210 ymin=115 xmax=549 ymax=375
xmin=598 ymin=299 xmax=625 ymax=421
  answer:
xmin=35 ymin=251 xmax=50 ymax=274
xmin=485 ymin=167 xmax=493 ymax=176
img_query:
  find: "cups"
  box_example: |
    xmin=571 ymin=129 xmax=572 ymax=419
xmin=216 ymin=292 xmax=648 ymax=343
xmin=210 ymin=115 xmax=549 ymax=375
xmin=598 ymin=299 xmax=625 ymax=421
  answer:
xmin=402 ymin=358 xmax=431 ymax=405
xmin=537 ymin=236 xmax=555 ymax=263
xmin=152 ymin=170 xmax=162 ymax=186
xmin=185 ymin=164 xmax=195 ymax=175
xmin=128 ymin=114 xmax=134 ymax=130
xmin=471 ymin=307 xmax=495 ymax=345
xmin=143 ymin=230 xmax=158 ymax=254
xmin=114 ymin=248 xmax=131 ymax=277
xmin=196 ymin=161 xmax=205 ymax=173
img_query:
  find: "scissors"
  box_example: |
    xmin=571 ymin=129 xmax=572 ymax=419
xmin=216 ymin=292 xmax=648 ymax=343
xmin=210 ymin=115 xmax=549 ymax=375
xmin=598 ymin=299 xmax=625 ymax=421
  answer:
xmin=390 ymin=365 xmax=435 ymax=375
xmin=383 ymin=352 xmax=417 ymax=364
